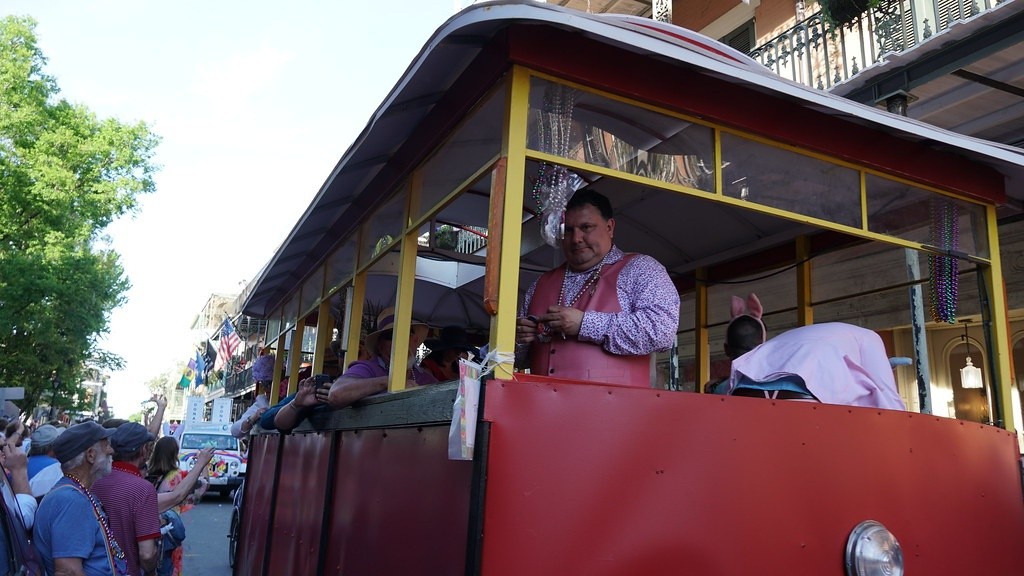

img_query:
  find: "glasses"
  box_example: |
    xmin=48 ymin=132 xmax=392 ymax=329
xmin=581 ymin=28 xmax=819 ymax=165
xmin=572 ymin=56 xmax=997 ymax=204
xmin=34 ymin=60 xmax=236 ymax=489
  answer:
xmin=336 ymin=349 xmax=362 ymax=359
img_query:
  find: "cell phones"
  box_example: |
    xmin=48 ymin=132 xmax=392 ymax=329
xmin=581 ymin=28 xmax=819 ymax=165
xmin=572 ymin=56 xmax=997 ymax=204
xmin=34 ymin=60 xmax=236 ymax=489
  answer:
xmin=314 ymin=374 xmax=333 ymax=399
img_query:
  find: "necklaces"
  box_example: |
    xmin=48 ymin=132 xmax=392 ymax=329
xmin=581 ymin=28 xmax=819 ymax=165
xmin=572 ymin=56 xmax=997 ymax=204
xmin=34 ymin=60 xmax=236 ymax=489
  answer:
xmin=559 ymin=252 xmax=609 ymax=308
xmin=533 ymin=82 xmax=576 ymax=237
xmin=929 ymin=193 xmax=960 ymax=325
xmin=113 ymin=466 xmax=142 ymax=477
xmin=64 ymin=474 xmax=125 ymax=559
xmin=386 ymin=364 xmax=415 ymax=381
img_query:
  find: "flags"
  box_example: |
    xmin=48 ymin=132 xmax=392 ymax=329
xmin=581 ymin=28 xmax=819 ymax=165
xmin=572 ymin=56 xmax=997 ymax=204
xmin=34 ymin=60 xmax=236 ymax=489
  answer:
xmin=214 ymin=318 xmax=241 ymax=371
xmin=195 ymin=350 xmax=206 ymax=388
xmin=179 ymin=359 xmax=197 ymax=388
xmin=204 ymin=341 xmax=217 ymax=386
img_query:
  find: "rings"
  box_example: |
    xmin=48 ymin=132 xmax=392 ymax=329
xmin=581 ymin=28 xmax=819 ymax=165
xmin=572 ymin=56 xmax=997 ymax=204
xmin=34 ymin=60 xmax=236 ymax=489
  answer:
xmin=260 ymin=411 xmax=263 ymax=415
xmin=305 ymin=382 xmax=309 ymax=384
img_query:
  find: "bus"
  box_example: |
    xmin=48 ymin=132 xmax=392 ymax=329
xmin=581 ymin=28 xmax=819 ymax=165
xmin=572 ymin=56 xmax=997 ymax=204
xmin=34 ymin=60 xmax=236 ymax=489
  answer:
xmin=236 ymin=0 xmax=1024 ymax=576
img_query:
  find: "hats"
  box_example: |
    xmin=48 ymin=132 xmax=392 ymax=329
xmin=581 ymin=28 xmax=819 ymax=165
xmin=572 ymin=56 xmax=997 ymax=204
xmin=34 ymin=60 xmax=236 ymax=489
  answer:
xmin=111 ymin=421 xmax=155 ymax=452
xmin=364 ymin=306 xmax=429 ymax=357
xmin=330 ymin=327 xmax=368 ymax=353
xmin=52 ymin=421 xmax=117 ymax=463
xmin=31 ymin=425 xmax=66 ymax=448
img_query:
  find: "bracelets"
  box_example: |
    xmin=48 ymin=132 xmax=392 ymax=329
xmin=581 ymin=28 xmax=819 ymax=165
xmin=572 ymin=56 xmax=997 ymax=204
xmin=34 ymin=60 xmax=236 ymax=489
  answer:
xmin=290 ymin=402 xmax=296 ymax=411
xmin=247 ymin=416 xmax=253 ymax=425
xmin=14 ymin=432 xmax=22 ymax=435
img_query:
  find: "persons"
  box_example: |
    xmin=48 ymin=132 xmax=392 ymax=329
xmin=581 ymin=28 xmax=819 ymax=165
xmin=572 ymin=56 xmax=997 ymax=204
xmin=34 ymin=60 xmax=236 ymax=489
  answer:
xmin=515 ymin=188 xmax=681 ymax=387
xmin=0 ymin=394 xmax=215 ymax=576
xmin=241 ymin=439 xmax=249 ymax=458
xmin=230 ymin=308 xmax=474 ymax=437
xmin=704 ymin=315 xmax=767 ymax=392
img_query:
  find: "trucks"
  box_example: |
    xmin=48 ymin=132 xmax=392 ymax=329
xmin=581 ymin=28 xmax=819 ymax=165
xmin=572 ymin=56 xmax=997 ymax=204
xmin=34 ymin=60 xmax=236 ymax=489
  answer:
xmin=176 ymin=418 xmax=248 ymax=501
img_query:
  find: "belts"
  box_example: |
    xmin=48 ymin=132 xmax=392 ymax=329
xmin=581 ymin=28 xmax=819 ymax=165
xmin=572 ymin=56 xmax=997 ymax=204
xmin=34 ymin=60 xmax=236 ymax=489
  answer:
xmin=732 ymin=387 xmax=816 ymax=399
